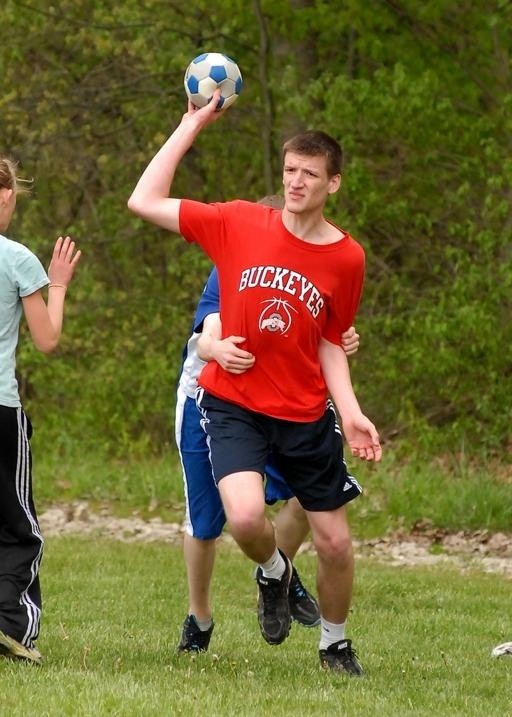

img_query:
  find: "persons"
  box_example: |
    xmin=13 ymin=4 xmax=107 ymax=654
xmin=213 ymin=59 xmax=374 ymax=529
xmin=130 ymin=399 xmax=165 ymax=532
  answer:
xmin=0 ymin=157 xmax=83 ymax=667
xmin=170 ymin=193 xmax=362 ymax=657
xmin=127 ymin=85 xmax=386 ymax=680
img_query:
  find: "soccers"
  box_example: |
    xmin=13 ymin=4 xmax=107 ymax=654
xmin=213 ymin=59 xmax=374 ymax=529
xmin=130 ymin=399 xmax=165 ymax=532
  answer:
xmin=183 ymin=53 xmax=242 ymax=113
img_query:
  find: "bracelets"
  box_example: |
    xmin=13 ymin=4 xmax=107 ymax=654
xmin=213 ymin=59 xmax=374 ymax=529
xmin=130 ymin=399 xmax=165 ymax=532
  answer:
xmin=47 ymin=281 xmax=67 ymax=292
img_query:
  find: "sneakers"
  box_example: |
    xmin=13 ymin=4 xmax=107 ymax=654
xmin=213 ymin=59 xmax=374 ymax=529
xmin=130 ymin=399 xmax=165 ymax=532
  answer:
xmin=319 ymin=639 xmax=364 ymax=677
xmin=255 ymin=547 xmax=320 ymax=645
xmin=177 ymin=615 xmax=214 ymax=653
xmin=0 ymin=629 xmax=45 ymax=666
